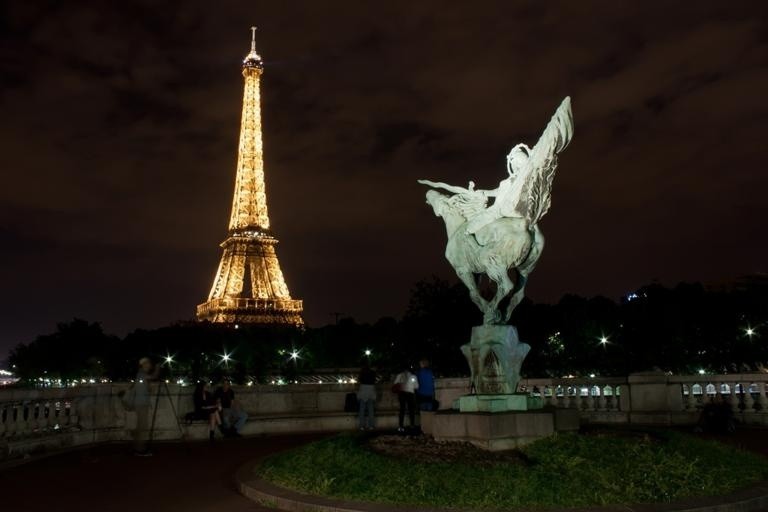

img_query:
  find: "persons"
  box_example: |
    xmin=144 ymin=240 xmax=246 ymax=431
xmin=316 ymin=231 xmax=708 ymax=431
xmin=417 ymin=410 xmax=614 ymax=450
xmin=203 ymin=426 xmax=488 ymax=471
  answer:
xmin=214 ymin=378 xmax=249 ymax=438
xmin=390 ymin=366 xmax=421 ymax=435
xmin=191 ymin=380 xmax=225 ymax=441
xmin=415 ymin=360 xmax=437 ymax=412
xmin=355 ymin=367 xmax=382 ymax=432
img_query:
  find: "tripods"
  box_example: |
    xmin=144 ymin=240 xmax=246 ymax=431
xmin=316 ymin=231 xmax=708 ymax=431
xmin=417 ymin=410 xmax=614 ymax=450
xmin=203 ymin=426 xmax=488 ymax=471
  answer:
xmin=148 ymin=379 xmax=187 ymax=446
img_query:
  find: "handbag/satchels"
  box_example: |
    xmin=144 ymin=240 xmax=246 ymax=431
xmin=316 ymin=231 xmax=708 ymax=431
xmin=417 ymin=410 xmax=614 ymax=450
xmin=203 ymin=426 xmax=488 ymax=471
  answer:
xmin=343 ymin=390 xmax=361 ymax=413
xmin=184 ymin=410 xmax=203 ymax=426
xmin=390 ymin=376 xmax=404 ymax=394
xmin=120 ymin=382 xmax=137 ymax=412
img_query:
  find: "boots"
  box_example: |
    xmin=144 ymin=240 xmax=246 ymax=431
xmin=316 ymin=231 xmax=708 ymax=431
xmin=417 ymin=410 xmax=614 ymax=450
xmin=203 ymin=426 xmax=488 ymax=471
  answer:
xmin=210 ymin=423 xmax=227 ymax=442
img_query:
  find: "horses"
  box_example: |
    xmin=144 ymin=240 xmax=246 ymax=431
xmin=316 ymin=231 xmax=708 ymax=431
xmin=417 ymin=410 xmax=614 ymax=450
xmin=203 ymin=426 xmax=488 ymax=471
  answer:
xmin=425 ymin=137 xmax=559 ymax=326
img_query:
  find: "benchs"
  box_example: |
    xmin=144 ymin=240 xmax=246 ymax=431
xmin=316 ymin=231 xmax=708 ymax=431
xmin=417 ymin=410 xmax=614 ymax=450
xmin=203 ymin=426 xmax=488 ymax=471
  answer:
xmin=185 ymin=407 xmax=583 ymax=440
